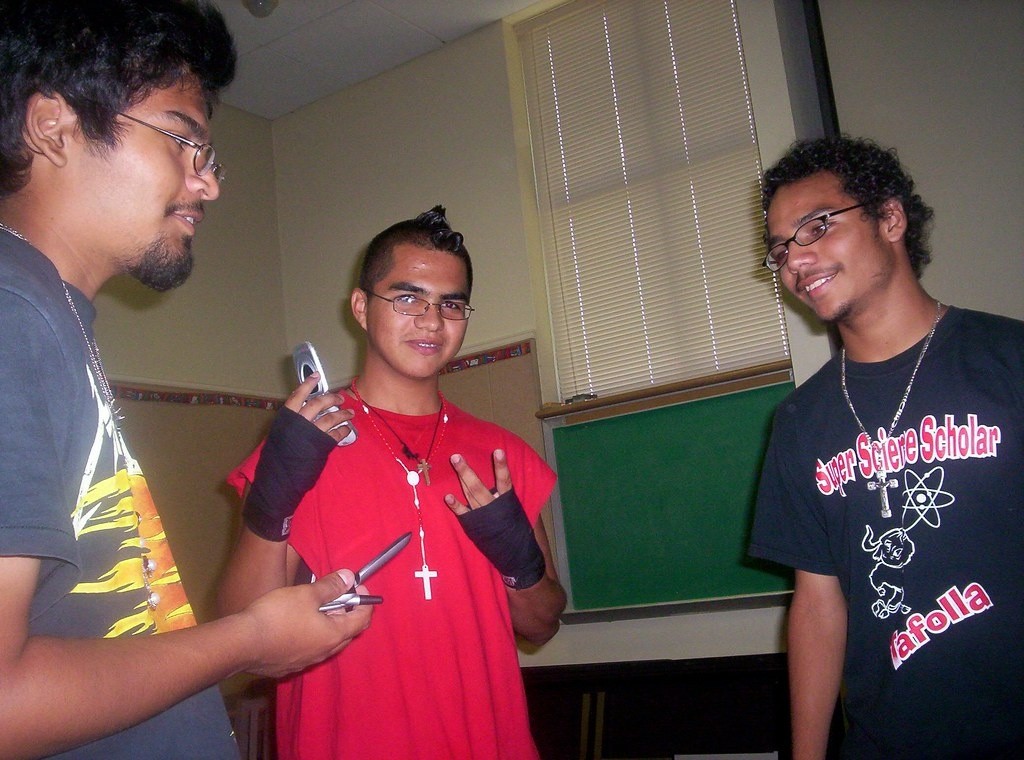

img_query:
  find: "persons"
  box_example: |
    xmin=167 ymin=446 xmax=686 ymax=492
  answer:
xmin=749 ymin=137 xmax=1024 ymax=759
xmin=220 ymin=204 xmax=567 ymax=760
xmin=0 ymin=0 xmax=376 ymax=760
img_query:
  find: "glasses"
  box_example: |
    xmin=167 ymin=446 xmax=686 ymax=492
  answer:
xmin=761 ymin=203 xmax=865 ymax=272
xmin=55 ymin=82 xmax=226 ymax=182
xmin=359 ymin=287 xmax=475 ymax=321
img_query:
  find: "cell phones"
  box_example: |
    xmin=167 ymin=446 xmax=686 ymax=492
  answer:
xmin=293 ymin=340 xmax=359 ymax=447
xmin=317 ymin=531 xmax=412 ymax=612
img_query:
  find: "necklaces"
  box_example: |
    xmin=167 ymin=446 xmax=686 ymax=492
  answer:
xmin=347 ymin=376 xmax=448 ymax=601
xmin=0 ymin=225 xmax=125 ymax=433
xmin=840 ymin=301 xmax=941 ymax=517
